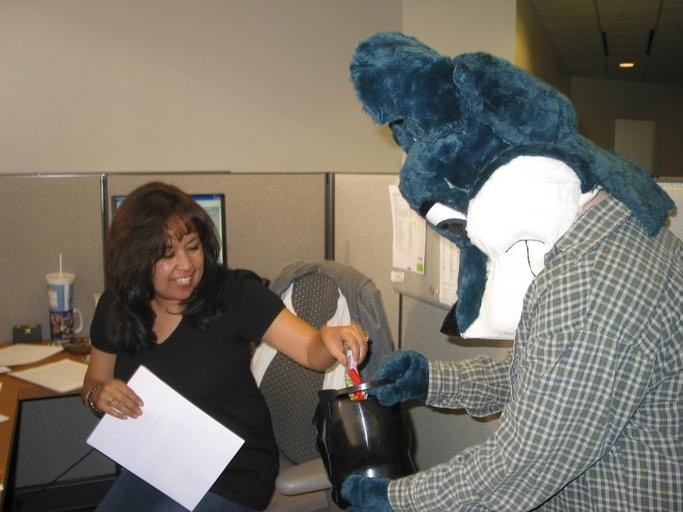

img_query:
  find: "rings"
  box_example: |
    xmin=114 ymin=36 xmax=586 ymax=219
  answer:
xmin=104 ymin=399 xmax=115 ymax=407
xmin=361 ymin=335 xmax=368 ymax=344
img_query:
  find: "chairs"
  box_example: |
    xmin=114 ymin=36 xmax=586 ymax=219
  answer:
xmin=247 ymin=259 xmax=396 ymax=512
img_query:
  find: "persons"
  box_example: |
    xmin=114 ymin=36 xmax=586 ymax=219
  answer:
xmin=77 ymin=182 xmax=368 ymax=512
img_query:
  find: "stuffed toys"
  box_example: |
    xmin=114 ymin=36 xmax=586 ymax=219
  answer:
xmin=336 ymin=30 xmax=683 ymax=512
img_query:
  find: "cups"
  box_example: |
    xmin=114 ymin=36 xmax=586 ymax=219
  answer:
xmin=45 ymin=307 xmax=83 ymax=343
xmin=45 ymin=273 xmax=74 ymax=312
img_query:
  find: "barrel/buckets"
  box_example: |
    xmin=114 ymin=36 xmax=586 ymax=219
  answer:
xmin=311 ymin=379 xmax=422 ymax=509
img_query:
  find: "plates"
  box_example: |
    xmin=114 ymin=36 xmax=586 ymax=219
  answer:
xmin=64 ymin=338 xmax=91 ymax=355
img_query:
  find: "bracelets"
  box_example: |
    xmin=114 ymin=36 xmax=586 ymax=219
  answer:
xmin=82 ymin=380 xmax=105 ymax=419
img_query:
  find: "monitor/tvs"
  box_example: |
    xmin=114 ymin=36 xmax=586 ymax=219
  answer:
xmin=110 ymin=191 xmax=229 ymax=271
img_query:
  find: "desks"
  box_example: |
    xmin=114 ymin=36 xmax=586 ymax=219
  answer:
xmin=0 ymin=335 xmax=126 ymax=510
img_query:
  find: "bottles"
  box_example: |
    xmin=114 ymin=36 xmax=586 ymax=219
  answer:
xmin=344 ymin=350 xmax=367 ymax=400
